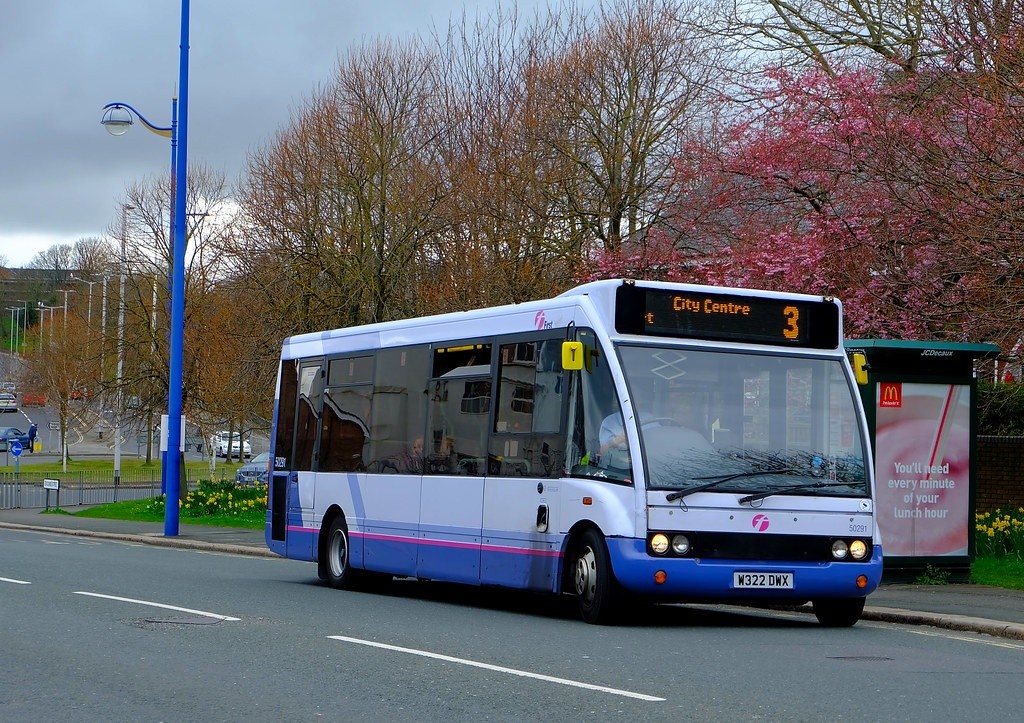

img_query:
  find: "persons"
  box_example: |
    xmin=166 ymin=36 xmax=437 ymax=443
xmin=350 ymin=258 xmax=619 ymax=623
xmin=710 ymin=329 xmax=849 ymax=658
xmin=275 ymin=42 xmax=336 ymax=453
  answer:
xmin=28 ymin=423 xmax=38 ymax=453
xmin=599 ymin=392 xmax=660 ymax=471
xmin=195 ymin=427 xmax=203 ymax=452
xmin=379 ymin=435 xmax=459 ymax=475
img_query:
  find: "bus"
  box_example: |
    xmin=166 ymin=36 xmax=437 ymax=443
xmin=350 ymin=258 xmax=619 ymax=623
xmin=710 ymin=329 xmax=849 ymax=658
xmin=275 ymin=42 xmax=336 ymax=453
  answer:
xmin=263 ymin=278 xmax=884 ymax=628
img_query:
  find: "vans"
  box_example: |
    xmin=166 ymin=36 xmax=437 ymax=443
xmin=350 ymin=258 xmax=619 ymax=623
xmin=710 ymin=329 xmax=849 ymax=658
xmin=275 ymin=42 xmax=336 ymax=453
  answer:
xmin=0 ymin=382 xmax=15 ymax=393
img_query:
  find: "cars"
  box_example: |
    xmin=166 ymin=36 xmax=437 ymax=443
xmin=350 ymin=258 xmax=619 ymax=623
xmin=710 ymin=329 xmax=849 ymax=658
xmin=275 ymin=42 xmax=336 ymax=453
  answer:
xmin=0 ymin=427 xmax=31 ymax=451
xmin=235 ymin=451 xmax=270 ymax=486
xmin=21 ymin=390 xmax=46 ymax=407
xmin=0 ymin=393 xmax=18 ymax=413
xmin=210 ymin=431 xmax=252 ymax=458
xmin=124 ymin=396 xmax=144 ymax=408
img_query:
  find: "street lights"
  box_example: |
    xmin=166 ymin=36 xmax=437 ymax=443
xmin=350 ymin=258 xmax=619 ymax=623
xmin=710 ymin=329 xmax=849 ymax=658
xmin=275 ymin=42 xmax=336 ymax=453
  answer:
xmin=113 ymin=203 xmax=136 ymax=485
xmin=99 ymin=101 xmax=188 ymax=537
xmin=5 ymin=273 xmax=114 ymax=390
